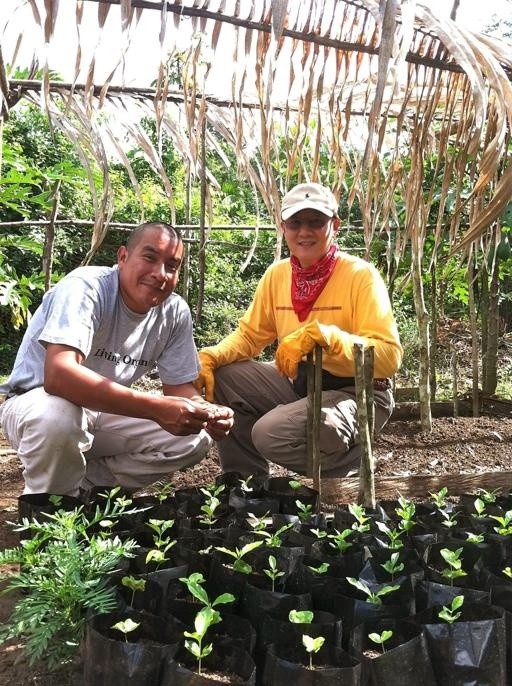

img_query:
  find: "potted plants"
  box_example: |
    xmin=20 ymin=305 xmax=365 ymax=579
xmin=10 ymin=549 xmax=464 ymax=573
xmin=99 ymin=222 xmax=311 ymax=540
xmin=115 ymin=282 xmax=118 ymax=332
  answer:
xmin=0 ymin=470 xmax=512 ymax=686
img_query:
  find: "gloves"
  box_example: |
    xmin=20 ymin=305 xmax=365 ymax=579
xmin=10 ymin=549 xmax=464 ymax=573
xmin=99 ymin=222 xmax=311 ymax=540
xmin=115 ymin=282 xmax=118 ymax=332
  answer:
xmin=274 ymin=318 xmax=329 ymax=381
xmin=192 ymin=352 xmax=218 ymax=404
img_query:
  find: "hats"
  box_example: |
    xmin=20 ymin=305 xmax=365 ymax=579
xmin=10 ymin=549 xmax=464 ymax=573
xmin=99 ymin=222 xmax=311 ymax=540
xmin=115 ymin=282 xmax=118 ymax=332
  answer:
xmin=280 ymin=182 xmax=339 ymax=223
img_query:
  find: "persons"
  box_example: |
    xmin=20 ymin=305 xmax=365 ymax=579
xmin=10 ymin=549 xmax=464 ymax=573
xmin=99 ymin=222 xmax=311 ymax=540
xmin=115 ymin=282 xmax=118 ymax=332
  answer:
xmin=193 ymin=182 xmax=404 ymax=481
xmin=0 ymin=222 xmax=236 ymax=511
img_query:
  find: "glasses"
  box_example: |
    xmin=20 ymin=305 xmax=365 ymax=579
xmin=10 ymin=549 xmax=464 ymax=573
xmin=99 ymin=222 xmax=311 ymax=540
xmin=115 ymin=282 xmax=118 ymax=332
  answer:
xmin=285 ymin=217 xmax=332 ymax=230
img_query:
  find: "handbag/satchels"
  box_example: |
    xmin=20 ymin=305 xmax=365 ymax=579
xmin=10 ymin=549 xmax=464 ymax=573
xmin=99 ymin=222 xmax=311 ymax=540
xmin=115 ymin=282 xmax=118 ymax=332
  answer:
xmin=293 ymin=360 xmax=391 ymax=398
